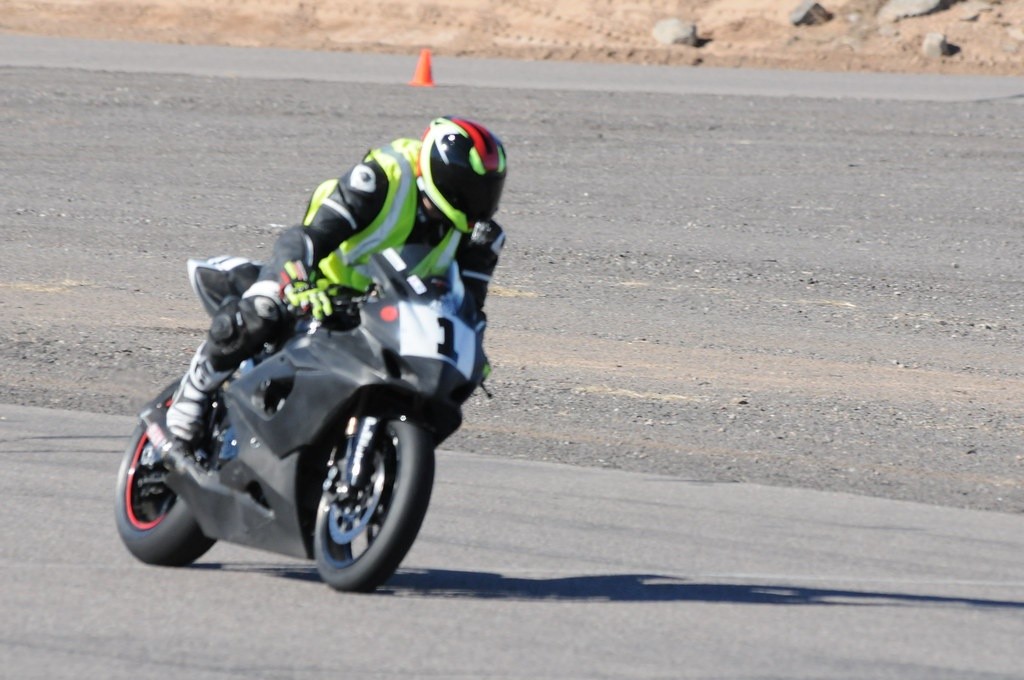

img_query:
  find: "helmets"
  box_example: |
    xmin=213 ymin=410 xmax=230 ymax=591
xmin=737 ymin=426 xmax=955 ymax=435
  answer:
xmin=417 ymin=117 xmax=507 ymax=233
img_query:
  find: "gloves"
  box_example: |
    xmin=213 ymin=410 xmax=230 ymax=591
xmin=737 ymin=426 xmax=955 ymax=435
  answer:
xmin=483 ymin=357 xmax=490 ymax=382
xmin=280 ymin=260 xmax=332 ymax=321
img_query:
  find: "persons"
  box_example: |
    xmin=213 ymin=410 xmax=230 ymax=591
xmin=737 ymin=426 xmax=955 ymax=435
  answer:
xmin=166 ymin=115 xmax=507 ymax=441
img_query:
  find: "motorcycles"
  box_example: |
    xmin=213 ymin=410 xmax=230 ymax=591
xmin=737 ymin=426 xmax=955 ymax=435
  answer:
xmin=114 ymin=245 xmax=492 ymax=593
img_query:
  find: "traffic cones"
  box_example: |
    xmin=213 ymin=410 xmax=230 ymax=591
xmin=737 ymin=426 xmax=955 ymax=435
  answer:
xmin=408 ymin=49 xmax=435 ymax=87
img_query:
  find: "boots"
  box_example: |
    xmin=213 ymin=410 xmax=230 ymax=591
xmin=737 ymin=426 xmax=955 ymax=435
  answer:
xmin=166 ymin=341 xmax=240 ymax=444
